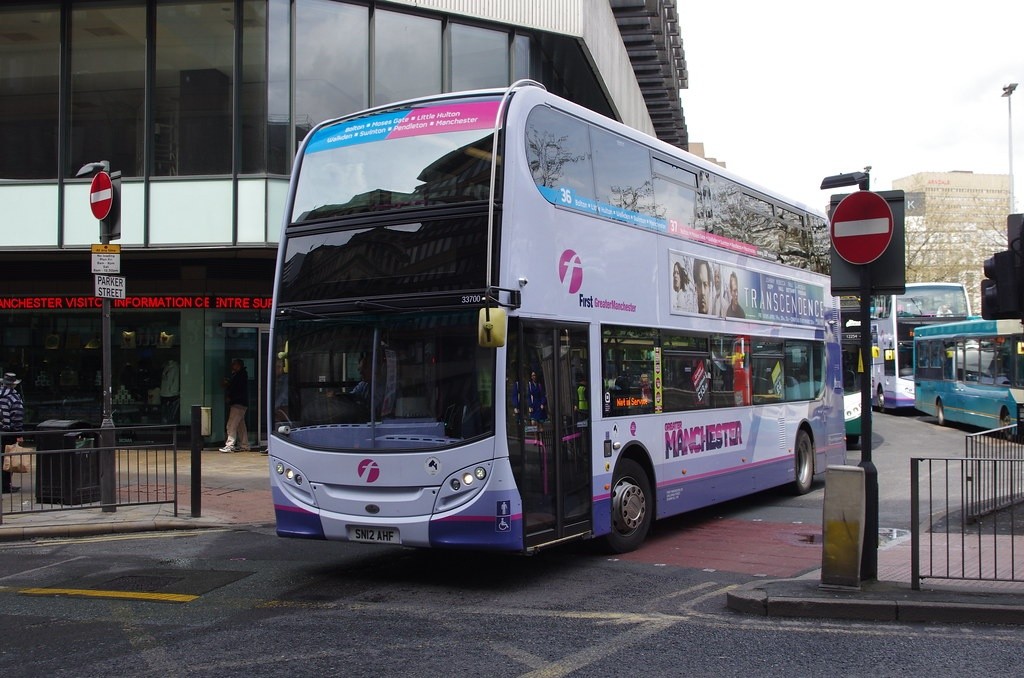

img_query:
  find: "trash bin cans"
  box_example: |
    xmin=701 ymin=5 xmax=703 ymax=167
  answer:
xmin=36 ymin=419 xmax=102 ymax=505
xmin=821 ymin=465 xmax=866 ymax=594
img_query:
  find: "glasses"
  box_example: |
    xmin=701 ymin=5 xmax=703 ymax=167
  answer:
xmin=232 ymin=363 xmax=236 ymax=365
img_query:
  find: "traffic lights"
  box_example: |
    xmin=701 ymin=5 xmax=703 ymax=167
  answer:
xmin=982 ymin=253 xmax=1011 ymax=318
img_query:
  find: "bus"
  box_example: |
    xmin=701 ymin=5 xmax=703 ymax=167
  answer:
xmin=840 ymin=300 xmax=864 ymax=449
xmin=913 ymin=319 xmax=1024 ymax=443
xmin=870 ymin=282 xmax=972 ymax=413
xmin=268 ymin=78 xmax=849 ymax=553
xmin=606 ymin=350 xmax=783 ymax=413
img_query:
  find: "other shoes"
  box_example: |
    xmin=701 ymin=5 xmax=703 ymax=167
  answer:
xmin=2 ymin=487 xmax=20 ymax=493
xmin=539 ymin=408 xmax=550 ymax=421
xmin=534 ymin=440 xmax=537 ymax=445
xmin=540 ymin=441 xmax=544 ymax=447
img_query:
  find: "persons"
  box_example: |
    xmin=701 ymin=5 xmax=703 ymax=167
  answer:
xmin=160 ymin=358 xmax=180 ymax=422
xmin=220 ymin=359 xmax=250 ymax=453
xmin=637 ymin=371 xmax=653 ymax=407
xmin=512 ymin=366 xmax=548 ymax=447
xmin=259 ymin=360 xmax=289 ymax=453
xmin=936 ymin=304 xmax=953 ymax=317
xmin=577 ymin=377 xmax=589 ymax=418
xmin=346 ymin=358 xmax=385 ymax=419
xmin=0 ymin=373 xmax=25 ymax=493
xmin=674 ymin=258 xmax=746 ymax=320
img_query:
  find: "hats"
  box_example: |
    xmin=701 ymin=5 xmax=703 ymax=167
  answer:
xmin=233 ymin=359 xmax=244 ymax=367
xmin=0 ymin=373 xmax=22 ymax=385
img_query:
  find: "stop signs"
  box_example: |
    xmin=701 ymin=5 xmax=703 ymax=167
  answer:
xmin=831 ymin=189 xmax=893 ymax=265
xmin=89 ymin=172 xmax=113 ymax=220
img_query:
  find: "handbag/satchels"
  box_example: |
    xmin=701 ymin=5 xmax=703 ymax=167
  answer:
xmin=3 ymin=441 xmax=34 ymax=473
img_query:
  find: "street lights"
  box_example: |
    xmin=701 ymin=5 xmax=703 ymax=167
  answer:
xmin=1003 ymin=82 xmax=1018 ymax=216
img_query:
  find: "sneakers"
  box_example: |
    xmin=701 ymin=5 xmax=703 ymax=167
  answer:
xmin=219 ymin=447 xmax=231 ymax=453
xmin=235 ymin=446 xmax=250 ymax=451
